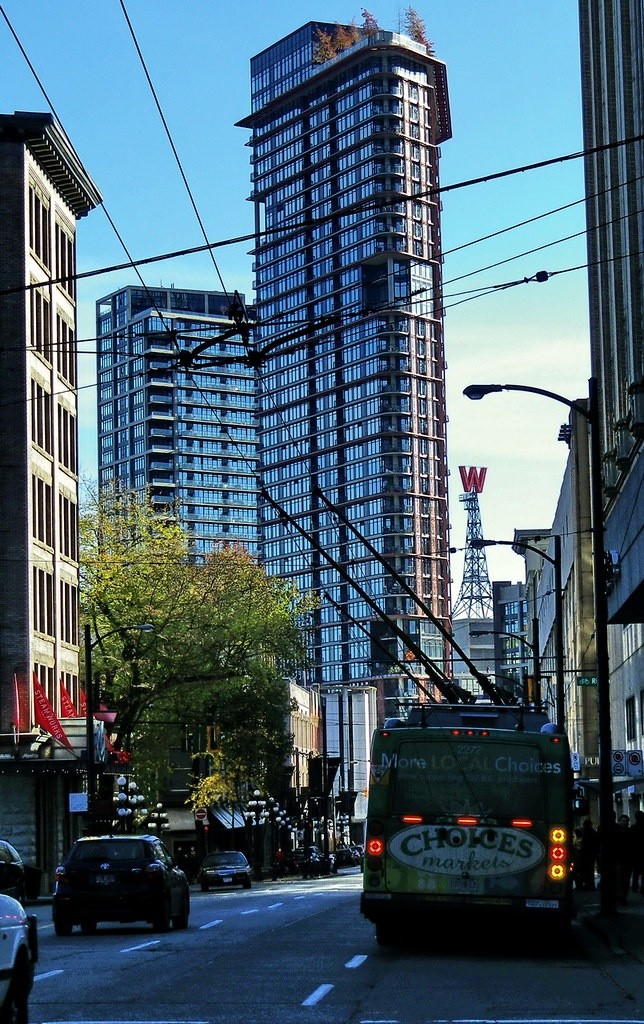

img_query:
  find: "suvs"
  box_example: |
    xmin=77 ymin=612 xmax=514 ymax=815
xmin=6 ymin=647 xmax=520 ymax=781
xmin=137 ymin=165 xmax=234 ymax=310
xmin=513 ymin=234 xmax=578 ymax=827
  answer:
xmin=0 ymin=893 xmax=39 ymax=1024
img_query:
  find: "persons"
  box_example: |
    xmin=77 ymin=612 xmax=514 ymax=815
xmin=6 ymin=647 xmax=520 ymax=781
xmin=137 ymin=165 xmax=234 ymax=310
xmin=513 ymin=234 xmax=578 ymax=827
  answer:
xmin=275 ymin=848 xmax=284 ymax=861
xmin=350 ymin=841 xmax=356 ymax=846
xmin=576 ymin=811 xmax=644 ymax=906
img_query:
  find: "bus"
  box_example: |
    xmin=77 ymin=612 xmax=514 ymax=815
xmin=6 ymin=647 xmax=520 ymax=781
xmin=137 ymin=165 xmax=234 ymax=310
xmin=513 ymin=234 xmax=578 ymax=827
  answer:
xmin=358 ymin=700 xmax=589 ymax=957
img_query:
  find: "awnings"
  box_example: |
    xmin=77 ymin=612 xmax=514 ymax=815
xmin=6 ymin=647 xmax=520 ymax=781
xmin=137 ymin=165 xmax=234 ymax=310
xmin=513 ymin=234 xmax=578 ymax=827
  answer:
xmin=208 ymin=806 xmax=264 ymax=831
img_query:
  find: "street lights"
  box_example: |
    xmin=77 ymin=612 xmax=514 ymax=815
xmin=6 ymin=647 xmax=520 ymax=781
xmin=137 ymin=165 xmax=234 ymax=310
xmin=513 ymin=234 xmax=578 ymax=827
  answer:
xmin=147 ymin=802 xmax=172 ymax=835
xmin=468 ymin=617 xmax=541 ymax=707
xmin=83 ymin=622 xmax=157 ymax=838
xmin=115 ymin=776 xmax=148 ymax=835
xmin=462 ymin=376 xmax=620 ymax=920
xmin=470 ymin=534 xmax=565 ymax=729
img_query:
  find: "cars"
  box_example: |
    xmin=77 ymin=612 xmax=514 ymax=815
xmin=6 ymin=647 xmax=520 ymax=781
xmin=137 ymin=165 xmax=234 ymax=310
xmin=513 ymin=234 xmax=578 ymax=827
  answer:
xmin=0 ymin=839 xmax=29 ymax=909
xmin=199 ymin=850 xmax=251 ymax=891
xmin=292 ymin=842 xmax=363 ymax=867
xmin=51 ymin=833 xmax=190 ymax=938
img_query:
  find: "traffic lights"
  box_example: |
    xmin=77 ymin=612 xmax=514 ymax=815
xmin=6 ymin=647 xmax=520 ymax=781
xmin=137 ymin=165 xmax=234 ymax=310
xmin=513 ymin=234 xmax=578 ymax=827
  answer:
xmin=244 ymin=791 xmax=298 ymax=870
